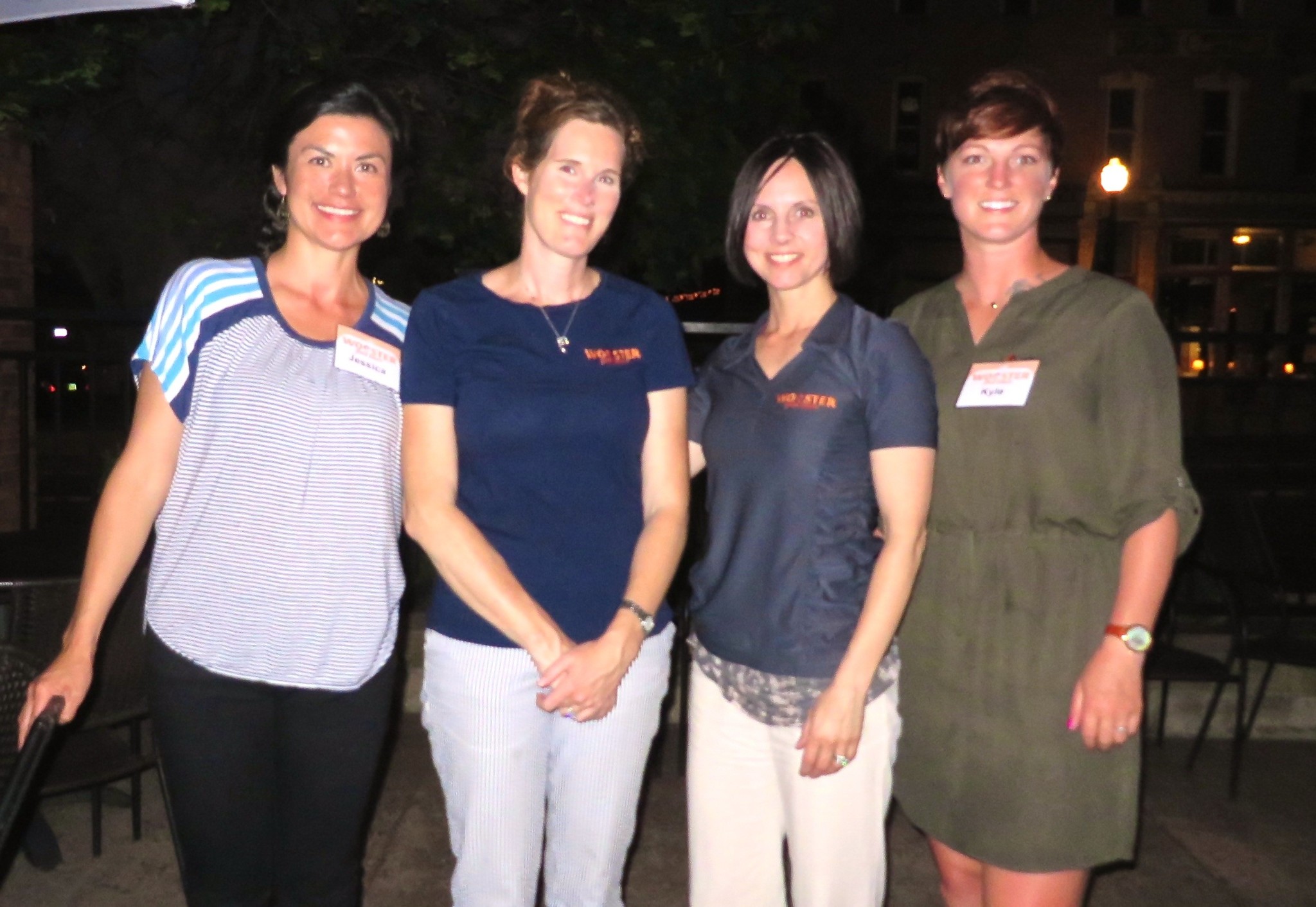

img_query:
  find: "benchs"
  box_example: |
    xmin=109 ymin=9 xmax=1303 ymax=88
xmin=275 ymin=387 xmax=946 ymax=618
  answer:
xmin=1 ymin=564 xmax=160 ymax=856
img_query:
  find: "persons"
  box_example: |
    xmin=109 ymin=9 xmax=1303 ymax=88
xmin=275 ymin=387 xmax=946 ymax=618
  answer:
xmin=400 ymin=68 xmax=698 ymax=907
xmin=11 ymin=74 xmax=430 ymax=906
xmin=685 ymin=132 xmax=937 ymax=907
xmin=876 ymin=70 xmax=1204 ymax=907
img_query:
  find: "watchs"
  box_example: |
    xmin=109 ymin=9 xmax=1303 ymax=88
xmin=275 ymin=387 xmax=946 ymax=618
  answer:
xmin=1104 ymin=621 xmax=1154 ymax=649
xmin=617 ymin=598 xmax=655 ymax=634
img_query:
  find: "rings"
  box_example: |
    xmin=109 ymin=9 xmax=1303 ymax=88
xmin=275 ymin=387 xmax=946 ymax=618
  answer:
xmin=835 ymin=754 xmax=849 ymax=766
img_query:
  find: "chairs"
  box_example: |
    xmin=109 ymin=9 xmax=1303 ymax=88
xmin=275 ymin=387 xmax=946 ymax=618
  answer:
xmin=1136 ymin=488 xmax=1246 ymax=799
xmin=1187 ymin=459 xmax=1316 ymax=793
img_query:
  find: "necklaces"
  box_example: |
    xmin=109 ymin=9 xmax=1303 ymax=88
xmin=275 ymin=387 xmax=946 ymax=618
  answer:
xmin=518 ymin=257 xmax=587 ymax=354
